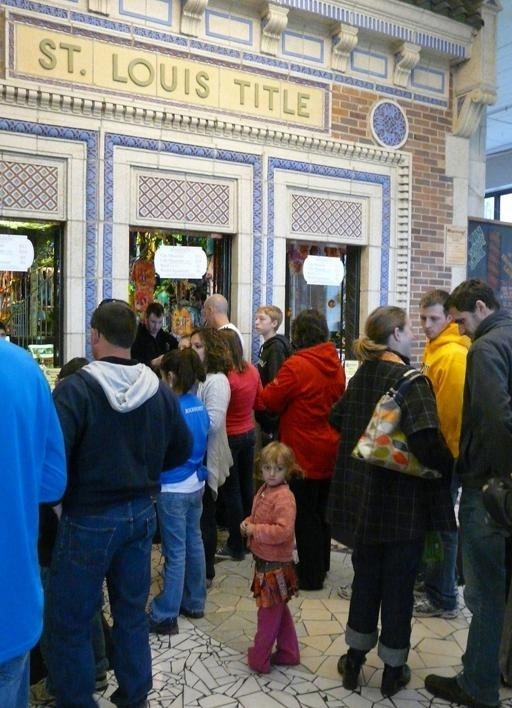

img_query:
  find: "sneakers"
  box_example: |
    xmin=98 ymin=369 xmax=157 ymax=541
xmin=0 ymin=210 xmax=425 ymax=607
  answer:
xmin=412 ymin=597 xmax=459 ymax=618
xmin=94 ymin=674 xmax=108 ymax=691
xmin=425 ymin=674 xmax=497 ymax=707
xmin=146 ymin=545 xmax=351 ymax=672
xmin=29 ymin=678 xmax=57 ymax=705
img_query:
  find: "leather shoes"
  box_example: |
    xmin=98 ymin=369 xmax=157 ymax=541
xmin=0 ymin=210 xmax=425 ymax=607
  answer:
xmin=338 ymin=654 xmax=366 ymax=689
xmin=381 ymin=663 xmax=410 ymax=697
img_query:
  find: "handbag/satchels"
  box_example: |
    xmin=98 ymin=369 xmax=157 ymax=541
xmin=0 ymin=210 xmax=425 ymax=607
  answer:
xmin=351 ymin=392 xmax=443 ymax=480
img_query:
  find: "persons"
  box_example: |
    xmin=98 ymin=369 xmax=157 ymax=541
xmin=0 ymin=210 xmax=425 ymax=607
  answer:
xmin=237 ymin=440 xmax=302 ymax=673
xmin=258 ymin=309 xmax=347 ymax=593
xmin=40 ymin=299 xmax=193 ymax=707
xmin=335 ymin=289 xmax=464 ymax=620
xmin=254 ymin=304 xmax=291 ymax=451
xmin=336 ymin=305 xmax=456 ymax=695
xmin=425 ymin=278 xmax=511 ymax=708
xmin=0 ymin=334 xmax=68 ymax=708
xmin=131 ymin=294 xmax=264 ymax=635
